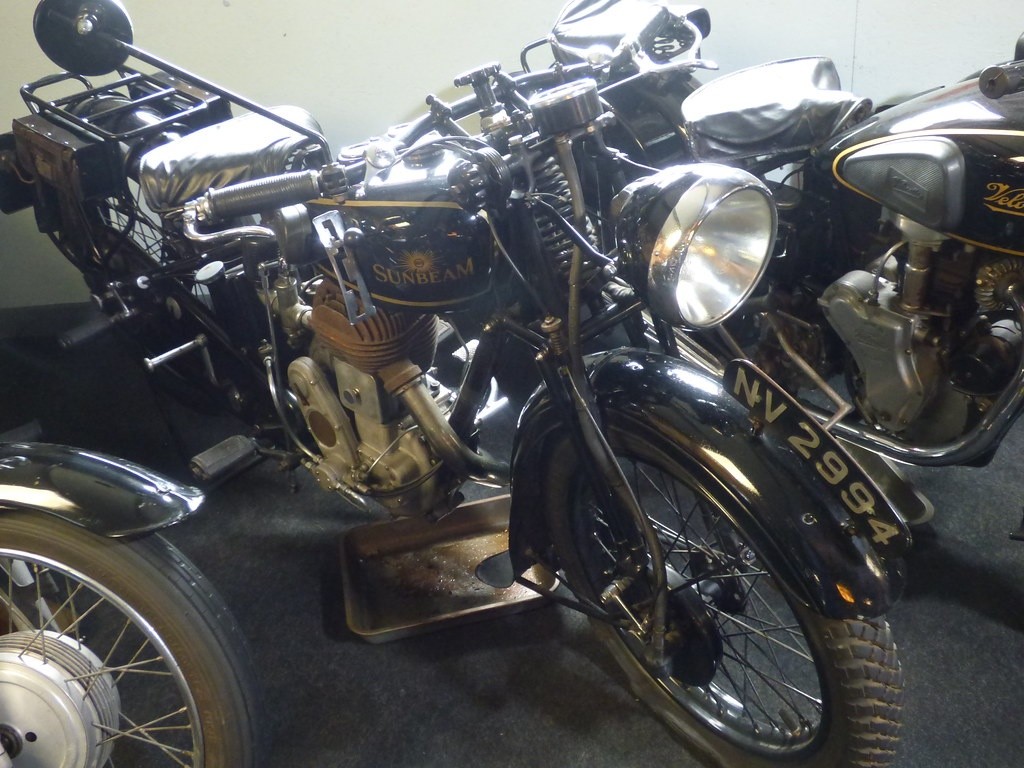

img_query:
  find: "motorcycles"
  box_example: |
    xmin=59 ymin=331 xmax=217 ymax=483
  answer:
xmin=0 ymin=439 xmax=272 ymax=768
xmin=337 ymin=0 xmax=1023 ymax=544
xmin=0 ymin=0 xmax=913 ymax=768
